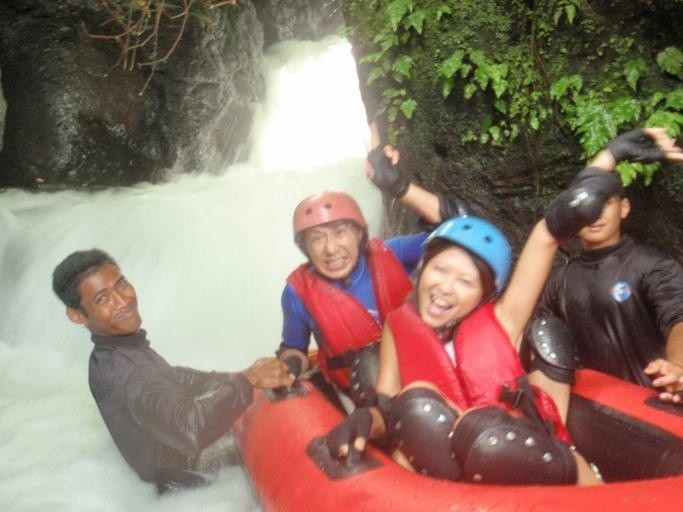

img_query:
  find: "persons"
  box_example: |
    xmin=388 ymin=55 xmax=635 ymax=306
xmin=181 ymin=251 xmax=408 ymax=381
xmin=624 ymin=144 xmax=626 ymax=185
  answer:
xmin=264 ymin=120 xmax=575 ymax=431
xmin=327 ymin=128 xmax=683 ymax=483
xmin=53 ymin=249 xmax=295 ymax=496
xmin=526 ymin=172 xmax=683 ymax=405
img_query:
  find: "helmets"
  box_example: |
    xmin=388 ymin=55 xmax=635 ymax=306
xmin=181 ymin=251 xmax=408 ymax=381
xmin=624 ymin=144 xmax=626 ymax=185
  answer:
xmin=421 ymin=215 xmax=515 ymax=295
xmin=293 ymin=191 xmax=369 ymax=246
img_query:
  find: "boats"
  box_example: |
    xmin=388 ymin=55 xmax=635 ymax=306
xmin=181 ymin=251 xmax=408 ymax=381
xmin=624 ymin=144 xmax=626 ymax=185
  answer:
xmin=233 ymin=350 xmax=683 ymax=512
